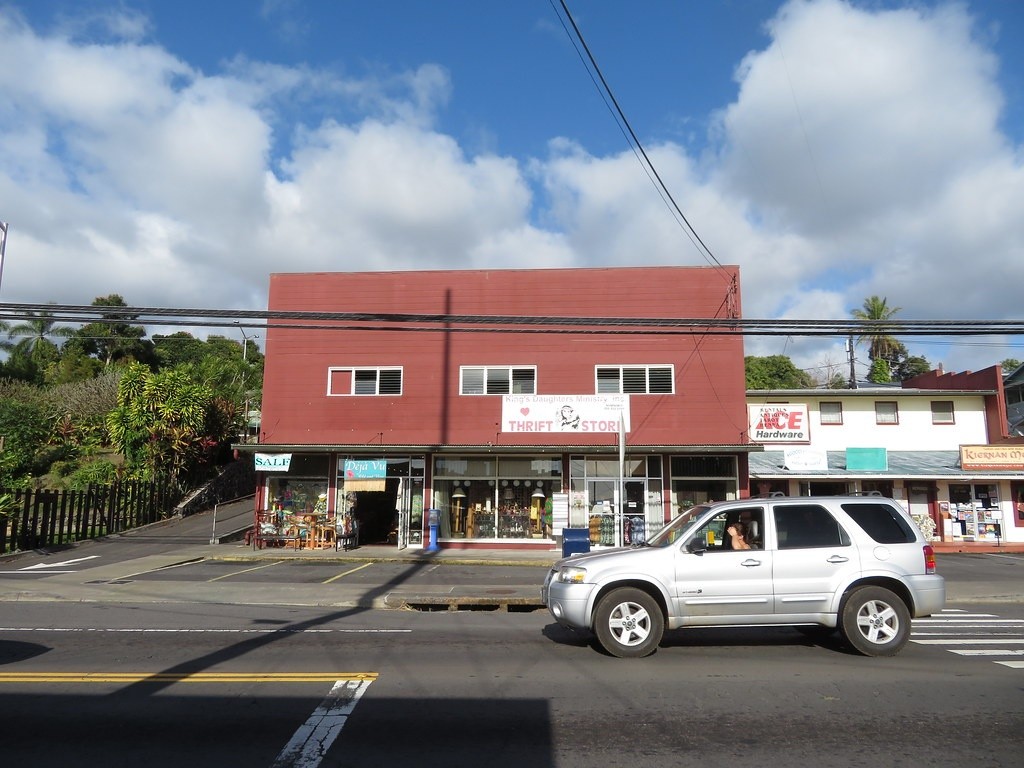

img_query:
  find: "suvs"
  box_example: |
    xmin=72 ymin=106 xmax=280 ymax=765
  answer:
xmin=540 ymin=489 xmax=946 ymax=659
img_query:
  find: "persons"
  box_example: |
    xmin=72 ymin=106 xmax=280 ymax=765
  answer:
xmin=727 ymin=522 xmax=753 ymax=551
xmin=555 ymin=405 xmax=580 ymax=432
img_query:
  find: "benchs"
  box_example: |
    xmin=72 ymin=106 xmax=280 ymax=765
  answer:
xmin=252 ymin=535 xmax=301 ymax=551
xmin=336 ymin=533 xmax=357 ymax=552
